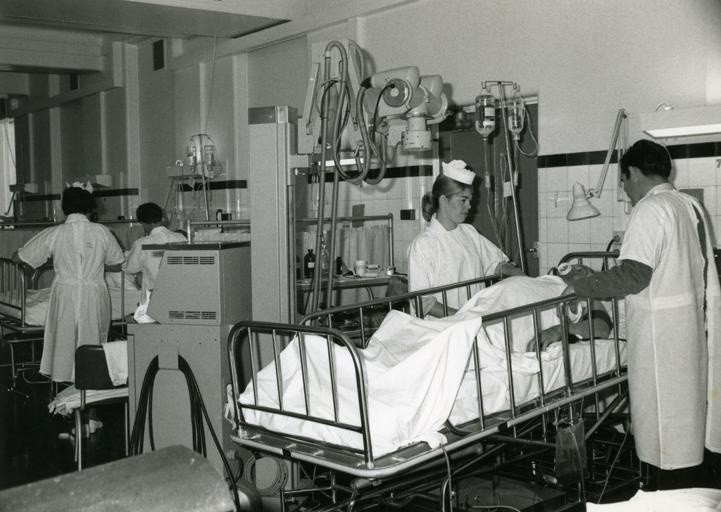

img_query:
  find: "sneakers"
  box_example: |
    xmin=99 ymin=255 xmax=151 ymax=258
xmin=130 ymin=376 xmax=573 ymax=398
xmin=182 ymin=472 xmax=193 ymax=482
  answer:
xmin=88 ymin=421 xmax=105 ymax=432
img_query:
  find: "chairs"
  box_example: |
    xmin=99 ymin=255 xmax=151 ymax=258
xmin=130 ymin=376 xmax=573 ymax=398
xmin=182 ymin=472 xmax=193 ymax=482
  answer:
xmin=61 ymin=338 xmax=131 ymax=473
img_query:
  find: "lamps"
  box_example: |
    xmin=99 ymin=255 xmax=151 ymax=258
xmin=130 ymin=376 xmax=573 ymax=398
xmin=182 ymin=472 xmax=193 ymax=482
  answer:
xmin=567 ymin=110 xmax=640 ymax=220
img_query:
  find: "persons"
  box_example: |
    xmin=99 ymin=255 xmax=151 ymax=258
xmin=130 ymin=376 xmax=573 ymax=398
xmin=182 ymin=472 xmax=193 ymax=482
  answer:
xmin=558 ymin=139 xmax=720 ymax=492
xmin=121 ymin=203 xmax=188 ymax=323
xmin=13 ymin=188 xmax=126 ymax=437
xmin=407 ymin=160 xmax=528 ymax=323
xmin=527 ymin=264 xmax=613 ymax=352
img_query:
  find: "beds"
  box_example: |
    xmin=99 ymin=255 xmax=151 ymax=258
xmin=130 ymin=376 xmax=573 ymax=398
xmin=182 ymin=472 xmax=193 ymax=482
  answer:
xmin=226 ymin=252 xmax=642 ymax=512
xmin=2 ymin=247 xmax=165 ymax=397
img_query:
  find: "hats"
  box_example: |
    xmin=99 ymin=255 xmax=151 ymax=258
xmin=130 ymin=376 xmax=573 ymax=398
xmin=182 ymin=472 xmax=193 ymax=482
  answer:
xmin=63 ymin=180 xmax=93 ymax=193
xmin=442 ymin=160 xmax=475 ymax=185
